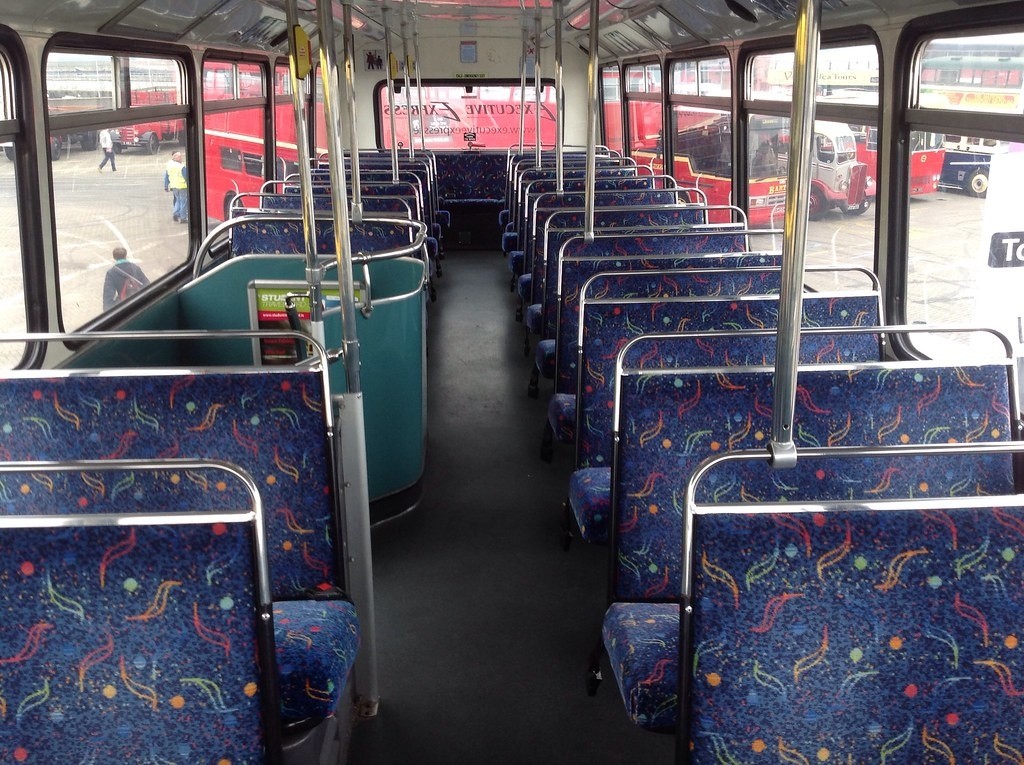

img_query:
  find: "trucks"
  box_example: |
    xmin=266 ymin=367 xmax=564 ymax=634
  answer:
xmin=3 ymin=103 xmax=105 ymax=163
xmin=110 ymin=86 xmax=187 ymax=154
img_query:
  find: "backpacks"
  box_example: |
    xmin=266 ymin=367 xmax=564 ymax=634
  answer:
xmin=120 ymin=277 xmax=141 ymax=301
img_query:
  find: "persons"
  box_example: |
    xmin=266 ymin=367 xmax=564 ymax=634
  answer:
xmin=97 ymin=129 xmax=117 ymax=173
xmin=103 ymin=247 xmax=151 ymax=311
xmin=164 ymin=151 xmax=188 ymax=224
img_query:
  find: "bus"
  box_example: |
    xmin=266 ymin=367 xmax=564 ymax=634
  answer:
xmin=780 ymin=42 xmax=1024 ymax=199
xmin=756 ymin=88 xmax=947 ymax=199
xmin=203 ymin=59 xmax=790 ymax=232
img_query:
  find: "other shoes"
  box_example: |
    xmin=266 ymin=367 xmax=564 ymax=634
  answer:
xmin=182 ymin=219 xmax=188 ymax=224
xmin=173 ymin=215 xmax=178 ymax=222
xmin=98 ymin=167 xmax=103 ymax=173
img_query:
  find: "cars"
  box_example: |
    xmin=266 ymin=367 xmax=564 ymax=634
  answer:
xmin=809 ymin=121 xmax=879 ymax=219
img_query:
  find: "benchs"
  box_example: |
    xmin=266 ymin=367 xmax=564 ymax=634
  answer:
xmin=0 ymin=141 xmax=1024 ymax=765
